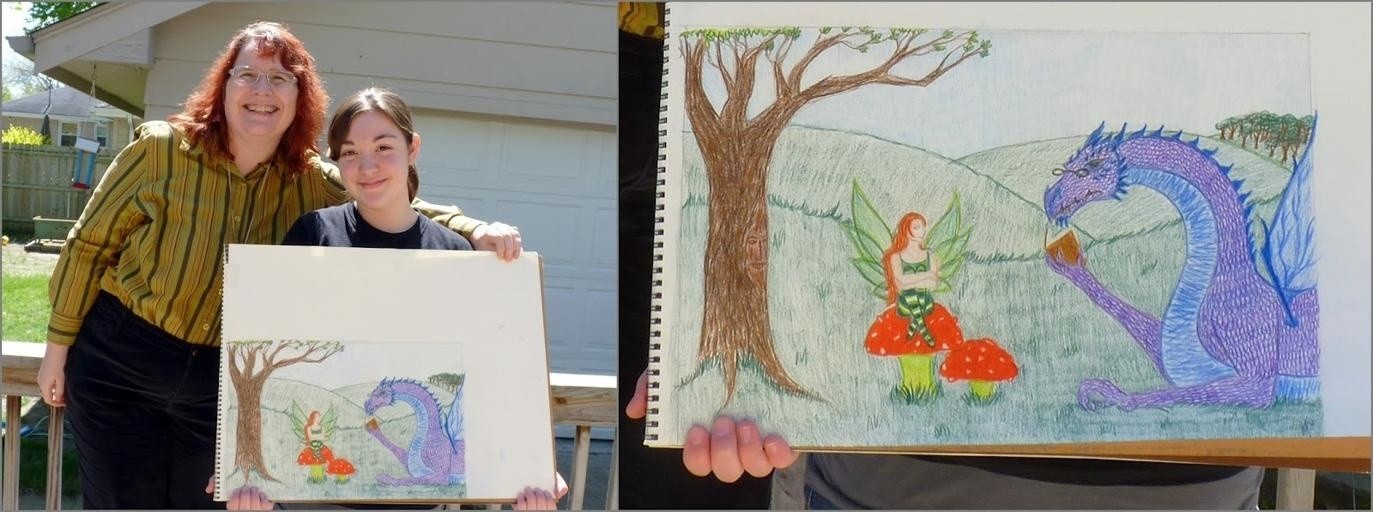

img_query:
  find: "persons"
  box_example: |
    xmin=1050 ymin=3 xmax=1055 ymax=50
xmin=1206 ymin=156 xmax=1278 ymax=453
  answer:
xmin=199 ymin=83 xmax=574 ymax=511
xmin=879 ymin=212 xmax=941 ymax=348
xmin=32 ymin=17 xmax=524 ymax=509
xmin=302 ymin=410 xmax=327 ymax=463
xmin=739 ymin=222 xmax=769 ymax=289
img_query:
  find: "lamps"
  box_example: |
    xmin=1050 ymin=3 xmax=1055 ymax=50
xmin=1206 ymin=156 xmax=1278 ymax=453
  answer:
xmin=38 ymin=78 xmax=53 ymax=144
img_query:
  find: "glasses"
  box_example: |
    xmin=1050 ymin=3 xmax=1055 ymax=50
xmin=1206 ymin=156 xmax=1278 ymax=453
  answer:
xmin=228 ymin=65 xmax=299 ymax=89
